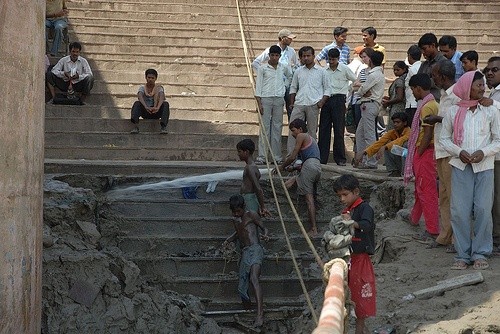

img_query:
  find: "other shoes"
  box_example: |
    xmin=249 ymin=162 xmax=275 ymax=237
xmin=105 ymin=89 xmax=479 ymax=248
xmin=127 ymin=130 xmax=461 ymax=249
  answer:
xmin=296 ymin=160 xmax=302 ymax=165
xmin=320 ymin=160 xmax=327 ymax=164
xmin=359 ymin=164 xmax=377 ymax=169
xmin=46 ymin=97 xmax=55 ymax=104
xmin=271 ymin=160 xmax=285 ymax=165
xmin=388 ymin=170 xmax=401 ymax=177
xmin=160 ymin=128 xmax=168 ymax=134
xmin=254 ymin=160 xmax=264 ymax=165
xmin=407 ymin=214 xmax=413 ymax=225
xmin=131 ymin=127 xmax=139 ymax=134
xmin=337 ymin=161 xmax=346 ymax=166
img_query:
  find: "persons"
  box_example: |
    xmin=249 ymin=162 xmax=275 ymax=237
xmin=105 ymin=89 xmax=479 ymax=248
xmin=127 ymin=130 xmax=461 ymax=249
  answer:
xmin=47 ymin=42 xmax=94 ymax=105
xmin=236 ymin=139 xmax=271 ymax=220
xmin=46 ymin=0 xmax=69 ymax=56
xmin=132 ymin=69 xmax=170 ymax=134
xmin=252 ymin=25 xmax=500 ymax=270
xmin=224 ymin=195 xmax=270 ymax=328
xmin=270 ymin=118 xmax=324 ymax=237
xmin=333 ymin=174 xmax=376 ymax=334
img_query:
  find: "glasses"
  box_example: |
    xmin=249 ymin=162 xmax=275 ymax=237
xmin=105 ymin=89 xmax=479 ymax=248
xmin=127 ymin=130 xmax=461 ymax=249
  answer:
xmin=485 ymin=67 xmax=500 ymax=72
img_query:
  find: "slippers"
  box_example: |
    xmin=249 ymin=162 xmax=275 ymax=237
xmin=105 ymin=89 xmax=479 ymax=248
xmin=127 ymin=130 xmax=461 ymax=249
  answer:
xmin=446 ymin=244 xmax=457 ymax=253
xmin=425 ymin=241 xmax=447 ymax=248
xmin=450 ymin=260 xmax=468 ymax=270
xmin=473 ymin=259 xmax=490 ymax=269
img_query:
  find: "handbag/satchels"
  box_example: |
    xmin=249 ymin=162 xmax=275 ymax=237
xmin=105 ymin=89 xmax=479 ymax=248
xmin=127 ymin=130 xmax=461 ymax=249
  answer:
xmin=345 ymin=90 xmax=356 ymax=133
xmin=54 ymin=92 xmax=81 ymax=105
